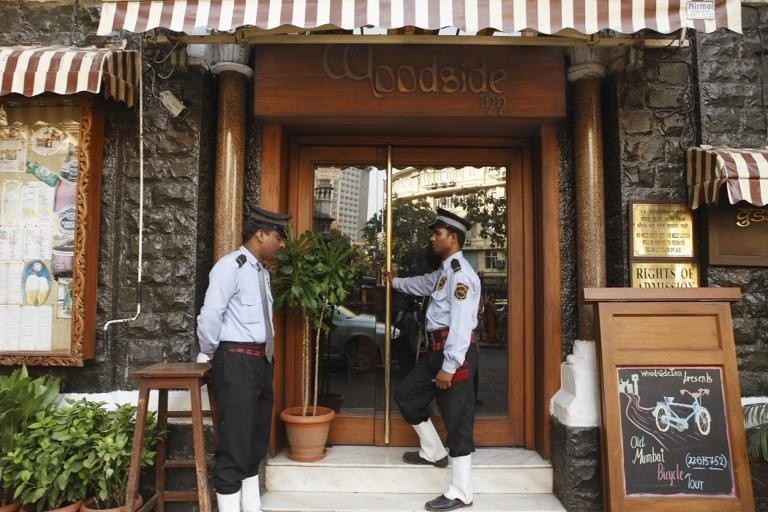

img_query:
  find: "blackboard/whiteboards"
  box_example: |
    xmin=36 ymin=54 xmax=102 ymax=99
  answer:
xmin=600 ymin=349 xmax=755 ymax=511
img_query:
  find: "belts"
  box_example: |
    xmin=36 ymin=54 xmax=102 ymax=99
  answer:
xmin=226 ymin=348 xmax=264 ymax=356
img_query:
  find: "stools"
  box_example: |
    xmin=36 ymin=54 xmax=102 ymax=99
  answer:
xmin=124 ymin=360 xmax=223 ymax=512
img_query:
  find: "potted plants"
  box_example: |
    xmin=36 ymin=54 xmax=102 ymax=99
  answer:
xmin=271 ymin=229 xmax=362 ymax=462
xmin=1 ymin=368 xmax=171 ymax=511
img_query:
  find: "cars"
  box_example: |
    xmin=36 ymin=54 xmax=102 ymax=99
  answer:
xmin=312 ymin=274 xmax=506 ymax=371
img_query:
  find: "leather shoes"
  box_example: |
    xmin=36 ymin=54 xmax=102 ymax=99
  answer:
xmin=423 ymin=495 xmax=473 ymax=512
xmin=402 ymin=451 xmax=447 ymax=468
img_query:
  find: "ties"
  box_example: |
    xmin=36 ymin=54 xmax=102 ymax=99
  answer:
xmin=254 ymin=261 xmax=273 ymax=365
xmin=423 ymin=270 xmax=444 ymax=353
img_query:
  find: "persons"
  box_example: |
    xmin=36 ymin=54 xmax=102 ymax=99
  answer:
xmin=194 ymin=204 xmax=295 ymax=512
xmin=381 ymin=205 xmax=485 ymax=512
xmin=392 ymin=293 xmax=424 ymax=381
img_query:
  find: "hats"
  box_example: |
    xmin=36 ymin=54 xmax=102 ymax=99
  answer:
xmin=248 ymin=205 xmax=292 ymax=237
xmin=427 ymin=208 xmax=470 ymax=234
